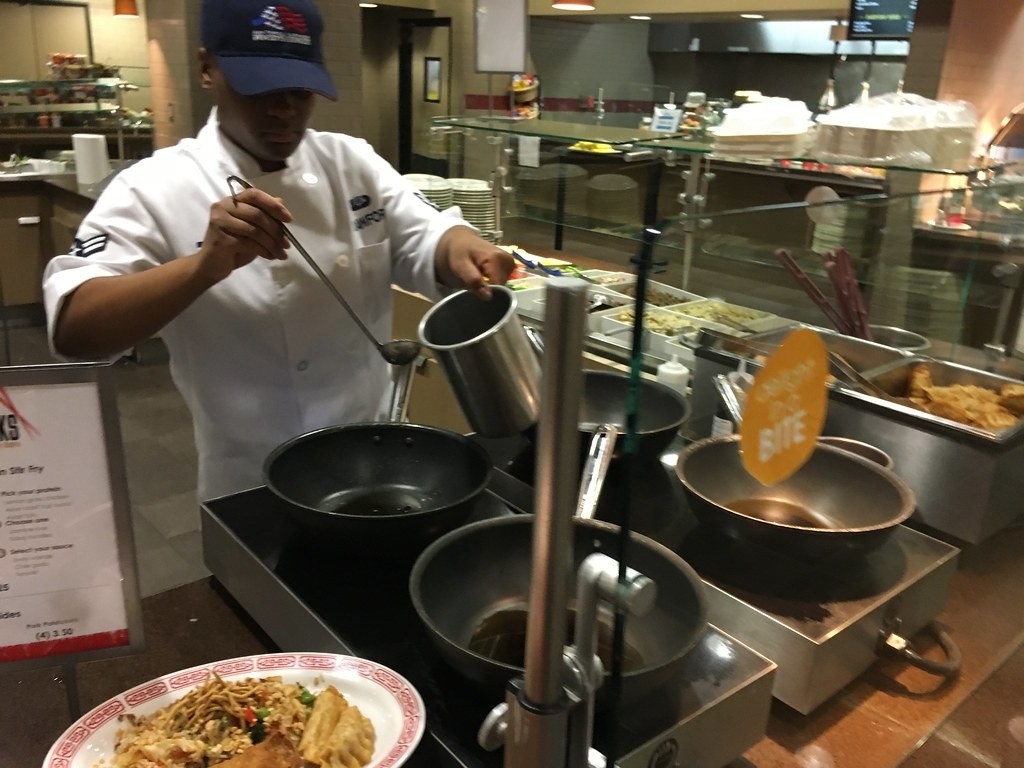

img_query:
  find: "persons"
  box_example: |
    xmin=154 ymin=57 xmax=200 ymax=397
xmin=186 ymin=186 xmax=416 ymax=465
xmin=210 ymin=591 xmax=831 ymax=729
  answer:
xmin=43 ymin=0 xmax=516 ymax=506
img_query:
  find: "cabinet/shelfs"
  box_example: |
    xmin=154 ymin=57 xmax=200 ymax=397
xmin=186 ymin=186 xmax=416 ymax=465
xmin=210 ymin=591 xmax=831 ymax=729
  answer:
xmin=45 ymin=183 xmax=97 ymax=262
xmin=0 ymin=181 xmax=47 ymax=327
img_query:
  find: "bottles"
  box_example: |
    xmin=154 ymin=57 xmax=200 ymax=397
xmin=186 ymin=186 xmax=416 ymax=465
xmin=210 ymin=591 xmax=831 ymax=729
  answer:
xmin=655 ymin=354 xmax=689 ymax=398
xmin=815 ymin=78 xmax=906 ymax=117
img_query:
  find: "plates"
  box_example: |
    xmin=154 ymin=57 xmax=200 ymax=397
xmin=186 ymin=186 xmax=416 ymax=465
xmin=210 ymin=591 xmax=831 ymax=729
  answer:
xmin=867 ymin=264 xmax=965 ymax=344
xmin=808 ymin=199 xmax=867 ymax=258
xmin=568 ymin=146 xmax=623 ymax=154
xmin=402 ymin=173 xmax=496 ymax=245
xmin=927 ymin=220 xmax=973 ymax=230
xmin=518 ymin=162 xmax=639 ymax=227
xmin=40 ymin=652 xmax=427 ymax=768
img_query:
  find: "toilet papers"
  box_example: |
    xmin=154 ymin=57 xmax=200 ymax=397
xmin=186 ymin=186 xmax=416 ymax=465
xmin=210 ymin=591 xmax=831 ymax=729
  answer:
xmin=71 ymin=134 xmax=115 ymax=184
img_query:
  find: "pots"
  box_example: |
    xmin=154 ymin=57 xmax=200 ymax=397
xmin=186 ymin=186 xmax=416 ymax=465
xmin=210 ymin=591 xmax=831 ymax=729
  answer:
xmin=408 ymin=422 xmax=710 ymax=698
xmin=261 ymin=361 xmax=495 ymax=541
xmin=676 ymin=374 xmax=919 ymax=572
xmin=523 ymin=323 xmax=691 ymax=466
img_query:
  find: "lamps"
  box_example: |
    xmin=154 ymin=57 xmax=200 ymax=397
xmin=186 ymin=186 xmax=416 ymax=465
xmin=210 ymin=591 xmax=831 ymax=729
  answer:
xmin=551 ymin=0 xmax=595 ymax=11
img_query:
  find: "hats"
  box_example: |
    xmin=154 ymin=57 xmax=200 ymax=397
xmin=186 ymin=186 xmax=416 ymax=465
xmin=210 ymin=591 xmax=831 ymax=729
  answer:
xmin=198 ymin=0 xmax=338 ymax=103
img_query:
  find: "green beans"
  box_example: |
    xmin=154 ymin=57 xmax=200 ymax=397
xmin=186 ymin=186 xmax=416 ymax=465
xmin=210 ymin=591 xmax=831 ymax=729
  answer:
xmin=302 ymin=694 xmax=313 ymax=704
xmin=257 ymin=708 xmax=267 ymax=719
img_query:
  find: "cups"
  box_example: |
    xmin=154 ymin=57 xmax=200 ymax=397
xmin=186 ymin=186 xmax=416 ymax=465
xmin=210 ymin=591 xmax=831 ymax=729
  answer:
xmin=862 ymin=324 xmax=932 ymax=354
xmin=36 ymin=116 xmax=49 ymax=128
xmin=51 ymin=116 xmax=62 ymax=127
xmin=417 ymin=284 xmax=544 ymax=438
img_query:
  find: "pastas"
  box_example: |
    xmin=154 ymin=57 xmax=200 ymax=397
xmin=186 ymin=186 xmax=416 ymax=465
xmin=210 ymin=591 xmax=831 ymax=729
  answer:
xmin=158 ymin=668 xmax=267 ymax=733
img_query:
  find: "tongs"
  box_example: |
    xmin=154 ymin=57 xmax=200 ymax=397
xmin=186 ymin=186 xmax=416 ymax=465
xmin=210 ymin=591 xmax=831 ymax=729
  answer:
xmin=825 ymin=351 xmax=925 ymax=413
